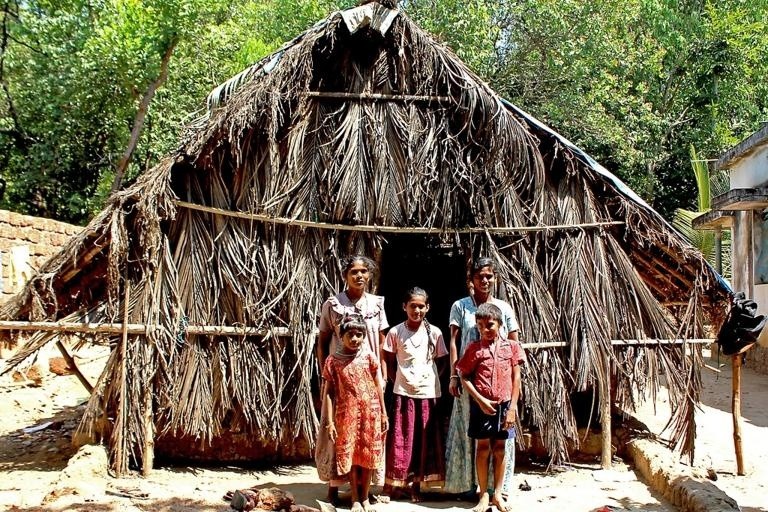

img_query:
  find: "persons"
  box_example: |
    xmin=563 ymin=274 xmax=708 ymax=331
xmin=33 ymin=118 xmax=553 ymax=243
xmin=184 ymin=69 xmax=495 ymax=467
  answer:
xmin=319 ymin=322 xmax=390 ymax=511
xmin=382 ymin=285 xmax=450 ymax=503
xmin=444 ymin=256 xmax=521 ymax=500
xmin=454 ymin=303 xmax=526 ymax=512
xmin=314 ymin=252 xmax=391 ymax=505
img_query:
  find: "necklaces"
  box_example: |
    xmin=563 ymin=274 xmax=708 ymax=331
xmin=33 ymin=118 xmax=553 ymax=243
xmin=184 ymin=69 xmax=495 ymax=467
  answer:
xmin=406 ymin=319 xmax=424 ymax=348
xmin=336 ymin=350 xmax=356 ymax=357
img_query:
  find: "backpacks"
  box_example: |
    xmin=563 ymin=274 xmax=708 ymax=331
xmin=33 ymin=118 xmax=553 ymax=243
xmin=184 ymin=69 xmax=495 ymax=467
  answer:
xmin=717 ymin=291 xmax=767 ymax=356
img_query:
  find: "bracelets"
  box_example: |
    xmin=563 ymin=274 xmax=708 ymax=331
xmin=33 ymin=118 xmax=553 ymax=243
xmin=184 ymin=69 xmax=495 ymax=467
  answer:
xmin=326 ymin=422 xmax=334 ymax=428
xmin=381 ymin=413 xmax=389 ymax=419
xmin=450 ymin=374 xmax=460 ymax=379
